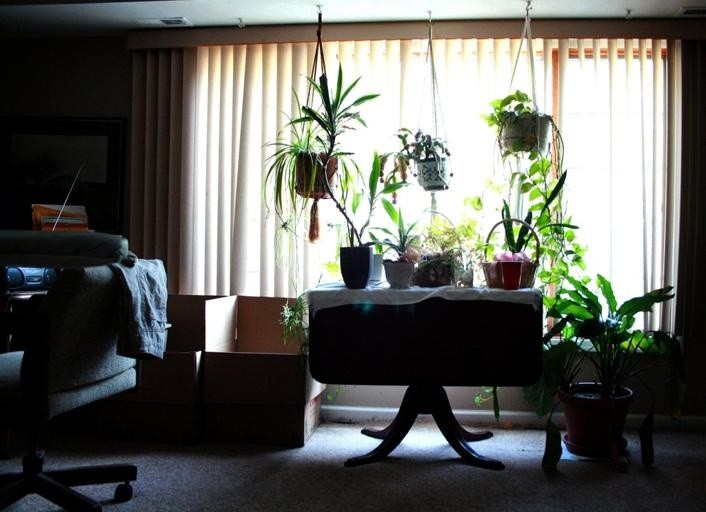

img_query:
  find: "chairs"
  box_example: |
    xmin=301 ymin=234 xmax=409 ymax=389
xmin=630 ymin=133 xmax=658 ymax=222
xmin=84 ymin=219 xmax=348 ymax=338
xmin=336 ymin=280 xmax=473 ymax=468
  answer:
xmin=0 ymin=259 xmax=143 ymax=511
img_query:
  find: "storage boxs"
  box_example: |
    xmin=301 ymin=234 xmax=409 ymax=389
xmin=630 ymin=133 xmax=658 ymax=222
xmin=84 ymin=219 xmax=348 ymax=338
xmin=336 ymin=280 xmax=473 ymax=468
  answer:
xmin=83 ymin=289 xmax=321 ymax=448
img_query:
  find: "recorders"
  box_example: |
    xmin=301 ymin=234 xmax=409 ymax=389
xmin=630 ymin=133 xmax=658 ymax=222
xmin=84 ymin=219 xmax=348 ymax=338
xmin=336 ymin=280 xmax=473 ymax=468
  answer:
xmin=4 ymin=264 xmax=57 ymax=289
